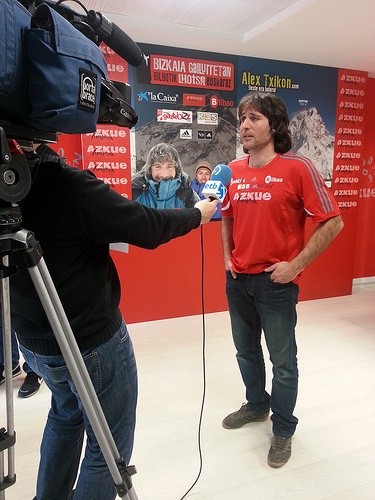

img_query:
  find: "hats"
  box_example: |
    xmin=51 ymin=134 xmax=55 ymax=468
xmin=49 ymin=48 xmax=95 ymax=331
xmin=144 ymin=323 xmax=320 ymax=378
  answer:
xmin=195 ymin=161 xmax=212 ymax=172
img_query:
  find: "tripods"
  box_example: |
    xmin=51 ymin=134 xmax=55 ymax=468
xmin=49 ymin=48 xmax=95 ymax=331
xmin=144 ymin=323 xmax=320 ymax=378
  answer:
xmin=1 ymin=155 xmax=139 ymax=500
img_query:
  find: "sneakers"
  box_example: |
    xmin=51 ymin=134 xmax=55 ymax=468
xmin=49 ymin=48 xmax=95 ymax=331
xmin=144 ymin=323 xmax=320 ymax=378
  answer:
xmin=18 ymin=371 xmax=43 ymax=398
xmin=222 ymin=402 xmax=270 ymax=429
xmin=0 ymin=361 xmax=22 ymax=385
xmin=268 ymin=436 xmax=292 ymax=468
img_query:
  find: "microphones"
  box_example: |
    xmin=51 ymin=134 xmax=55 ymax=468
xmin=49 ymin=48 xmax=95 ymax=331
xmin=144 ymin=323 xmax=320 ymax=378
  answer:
xmin=88 ymin=9 xmax=144 ymax=67
xmin=207 ymin=164 xmax=232 ymax=201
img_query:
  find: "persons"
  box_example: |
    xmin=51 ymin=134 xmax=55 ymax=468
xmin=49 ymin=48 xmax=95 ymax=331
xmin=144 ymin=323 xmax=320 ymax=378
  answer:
xmin=130 ymin=143 xmax=201 ymax=214
xmin=221 ymin=91 xmax=343 ymax=468
xmin=0 ymin=324 xmax=44 ymax=398
xmin=191 ymin=161 xmax=222 ymax=217
xmin=0 ymin=134 xmax=218 ymax=499
